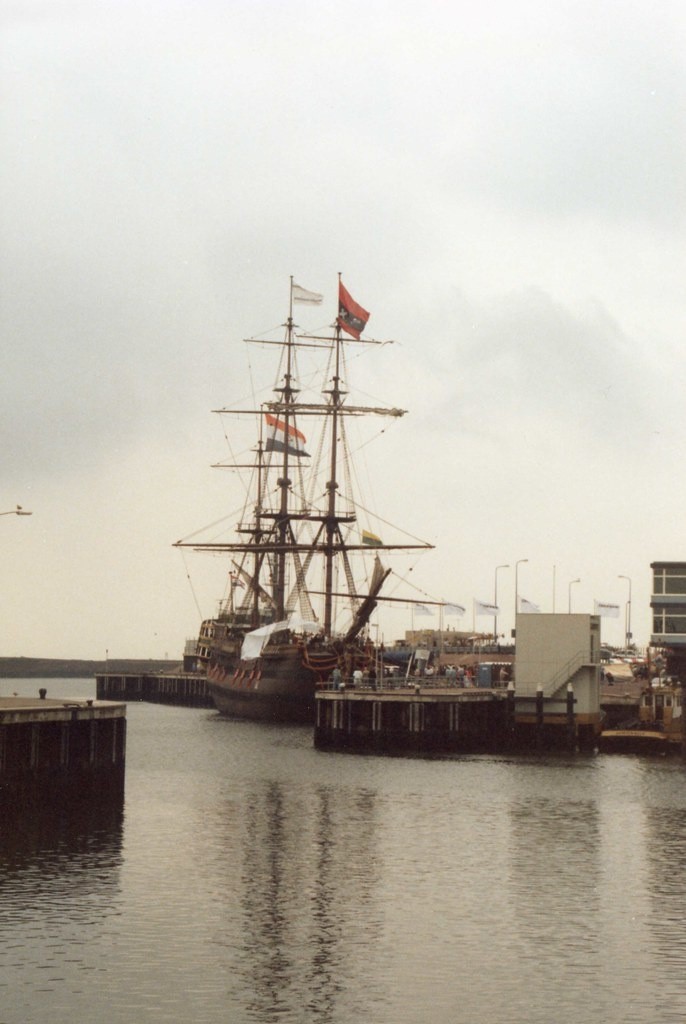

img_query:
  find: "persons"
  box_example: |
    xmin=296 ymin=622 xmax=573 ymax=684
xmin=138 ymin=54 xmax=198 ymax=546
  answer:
xmin=354 ymin=667 xmax=377 ymax=691
xmin=333 ymin=665 xmax=341 ymax=689
xmin=415 ymin=662 xmax=512 ymax=688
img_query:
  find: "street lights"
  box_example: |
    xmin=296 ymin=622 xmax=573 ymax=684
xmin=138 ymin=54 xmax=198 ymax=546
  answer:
xmin=569 ymin=579 xmax=581 ymax=613
xmin=618 ymin=575 xmax=632 ymax=647
xmin=515 ymin=559 xmax=528 ymax=613
xmin=494 ymin=565 xmax=510 ymax=643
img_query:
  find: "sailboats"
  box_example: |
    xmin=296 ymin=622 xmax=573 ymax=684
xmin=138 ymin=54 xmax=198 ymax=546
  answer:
xmin=172 ymin=272 xmax=448 ymax=723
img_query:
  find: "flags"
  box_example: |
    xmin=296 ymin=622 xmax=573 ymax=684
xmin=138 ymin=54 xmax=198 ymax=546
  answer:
xmin=362 ymin=530 xmax=382 ymax=546
xmin=264 ymin=413 xmax=311 ymax=457
xmin=337 ymin=282 xmax=370 ymax=339
xmin=292 ymin=284 xmax=322 ymax=306
